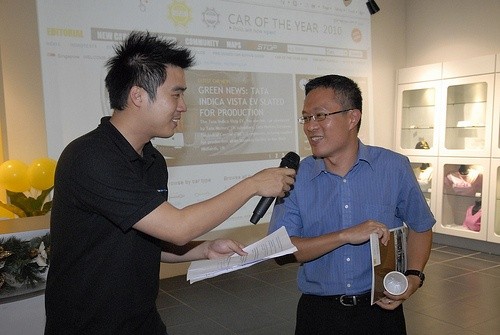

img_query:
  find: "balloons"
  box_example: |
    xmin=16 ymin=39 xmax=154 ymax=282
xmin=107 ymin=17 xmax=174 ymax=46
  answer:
xmin=0 ymin=157 xmax=57 ymax=198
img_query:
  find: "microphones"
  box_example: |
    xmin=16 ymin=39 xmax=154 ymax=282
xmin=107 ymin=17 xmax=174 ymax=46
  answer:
xmin=250 ymin=152 xmax=300 ymax=224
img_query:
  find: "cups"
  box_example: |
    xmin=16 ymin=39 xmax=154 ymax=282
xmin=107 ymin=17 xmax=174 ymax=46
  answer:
xmin=383 ymin=271 xmax=408 ymax=295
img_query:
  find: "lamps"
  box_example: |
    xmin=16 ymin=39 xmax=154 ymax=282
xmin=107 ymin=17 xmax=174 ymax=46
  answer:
xmin=366 ymin=0 xmax=380 ymax=15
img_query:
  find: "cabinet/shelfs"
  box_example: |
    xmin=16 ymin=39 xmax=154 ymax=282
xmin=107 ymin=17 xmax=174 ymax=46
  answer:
xmin=395 ymin=53 xmax=500 ymax=256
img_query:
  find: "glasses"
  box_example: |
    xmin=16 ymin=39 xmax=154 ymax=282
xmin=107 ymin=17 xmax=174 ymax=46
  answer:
xmin=297 ymin=108 xmax=353 ymax=124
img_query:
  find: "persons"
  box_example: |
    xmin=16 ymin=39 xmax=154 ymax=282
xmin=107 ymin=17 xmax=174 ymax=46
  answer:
xmin=414 ymin=163 xmax=483 ymax=232
xmin=267 ymin=75 xmax=436 ymax=335
xmin=44 ymin=31 xmax=295 ymax=335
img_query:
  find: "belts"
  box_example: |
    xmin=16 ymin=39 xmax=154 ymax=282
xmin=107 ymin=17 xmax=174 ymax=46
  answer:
xmin=325 ymin=292 xmax=371 ymax=307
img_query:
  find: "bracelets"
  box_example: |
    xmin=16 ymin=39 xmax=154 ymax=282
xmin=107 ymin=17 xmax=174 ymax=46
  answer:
xmin=405 ymin=270 xmax=425 ymax=287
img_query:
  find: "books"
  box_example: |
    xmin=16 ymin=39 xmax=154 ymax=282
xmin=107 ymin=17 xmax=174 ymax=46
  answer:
xmin=369 ymin=227 xmax=407 ymax=305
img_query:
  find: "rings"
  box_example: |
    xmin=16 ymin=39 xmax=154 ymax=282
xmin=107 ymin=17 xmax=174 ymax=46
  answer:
xmin=389 ymin=300 xmax=390 ymax=304
